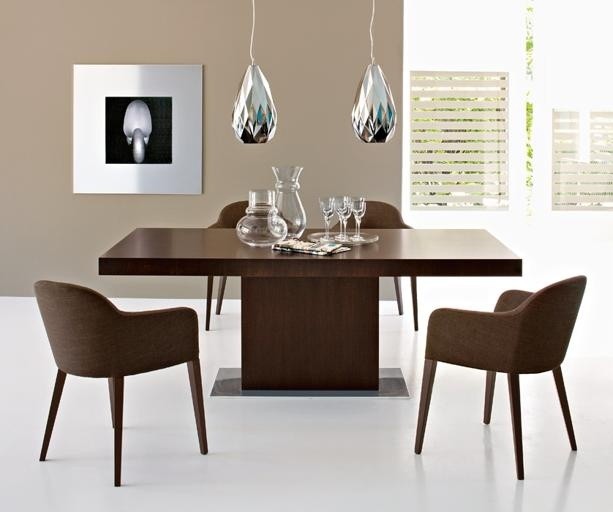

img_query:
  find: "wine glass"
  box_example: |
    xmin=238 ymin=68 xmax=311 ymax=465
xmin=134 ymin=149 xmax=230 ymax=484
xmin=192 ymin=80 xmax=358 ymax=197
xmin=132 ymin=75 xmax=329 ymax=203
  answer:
xmin=320 ymin=196 xmax=367 ymax=243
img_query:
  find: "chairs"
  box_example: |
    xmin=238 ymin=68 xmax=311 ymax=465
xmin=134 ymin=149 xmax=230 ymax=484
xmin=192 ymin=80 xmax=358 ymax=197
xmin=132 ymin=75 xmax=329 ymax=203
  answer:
xmin=328 ymin=201 xmax=419 ymax=331
xmin=205 ymin=200 xmax=300 ymax=331
xmin=414 ymin=276 xmax=586 ymax=480
xmin=35 ymin=280 xmax=208 ymax=486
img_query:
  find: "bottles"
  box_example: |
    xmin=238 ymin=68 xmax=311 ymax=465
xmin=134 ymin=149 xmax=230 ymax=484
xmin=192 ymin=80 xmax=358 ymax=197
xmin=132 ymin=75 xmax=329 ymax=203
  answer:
xmin=235 ymin=189 xmax=287 ymax=249
xmin=268 ymin=164 xmax=307 ymax=238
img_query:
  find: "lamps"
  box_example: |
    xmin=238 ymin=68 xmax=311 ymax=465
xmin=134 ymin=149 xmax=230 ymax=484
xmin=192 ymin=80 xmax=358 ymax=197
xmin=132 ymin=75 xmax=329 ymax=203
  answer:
xmin=231 ymin=1 xmax=278 ymax=145
xmin=351 ymin=1 xmax=398 ymax=144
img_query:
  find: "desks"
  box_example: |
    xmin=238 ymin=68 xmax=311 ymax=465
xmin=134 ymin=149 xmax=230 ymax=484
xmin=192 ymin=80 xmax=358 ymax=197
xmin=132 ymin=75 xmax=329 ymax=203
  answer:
xmin=99 ymin=228 xmax=522 ymax=398
